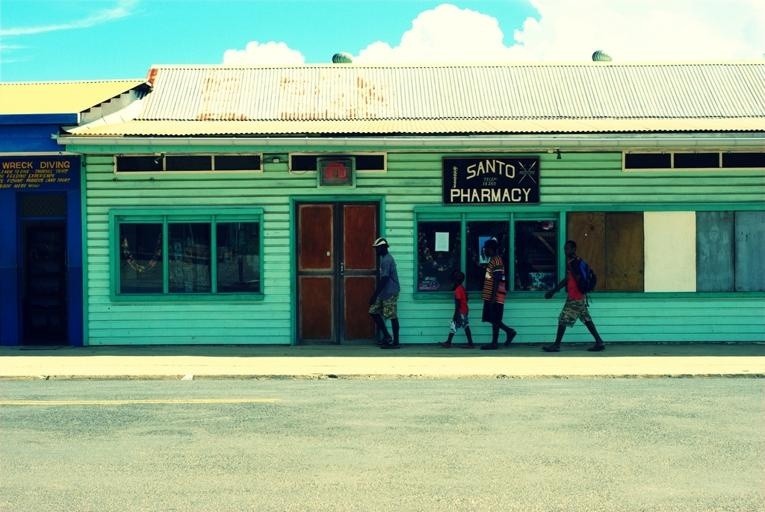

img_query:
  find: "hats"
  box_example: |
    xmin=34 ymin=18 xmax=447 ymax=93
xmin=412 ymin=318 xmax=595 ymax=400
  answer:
xmin=372 ymin=237 xmax=390 ymax=247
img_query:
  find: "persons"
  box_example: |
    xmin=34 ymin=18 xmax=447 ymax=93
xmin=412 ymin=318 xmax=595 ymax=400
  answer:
xmin=480 ymin=239 xmax=517 ymax=351
xmin=543 ymin=240 xmax=606 ymax=352
xmin=438 ymin=271 xmax=474 ymax=349
xmin=366 ymin=237 xmax=400 ymax=348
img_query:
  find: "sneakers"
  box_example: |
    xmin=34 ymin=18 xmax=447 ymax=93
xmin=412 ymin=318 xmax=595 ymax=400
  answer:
xmin=436 ymin=341 xmax=451 ymax=348
xmin=459 ymin=343 xmax=475 ymax=349
xmin=502 ymin=328 xmax=517 ymax=347
xmin=479 ymin=342 xmax=500 ymax=350
xmin=541 ymin=345 xmax=561 ymax=352
xmin=377 ymin=337 xmax=401 ymax=350
xmin=587 ymin=341 xmax=605 ymax=352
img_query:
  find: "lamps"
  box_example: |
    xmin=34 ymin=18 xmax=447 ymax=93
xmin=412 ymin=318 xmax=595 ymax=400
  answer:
xmin=153 ymin=152 xmax=167 ymax=165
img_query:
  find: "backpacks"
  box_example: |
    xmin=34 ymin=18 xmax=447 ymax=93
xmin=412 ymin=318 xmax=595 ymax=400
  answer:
xmin=572 ymin=257 xmax=598 ymax=296
